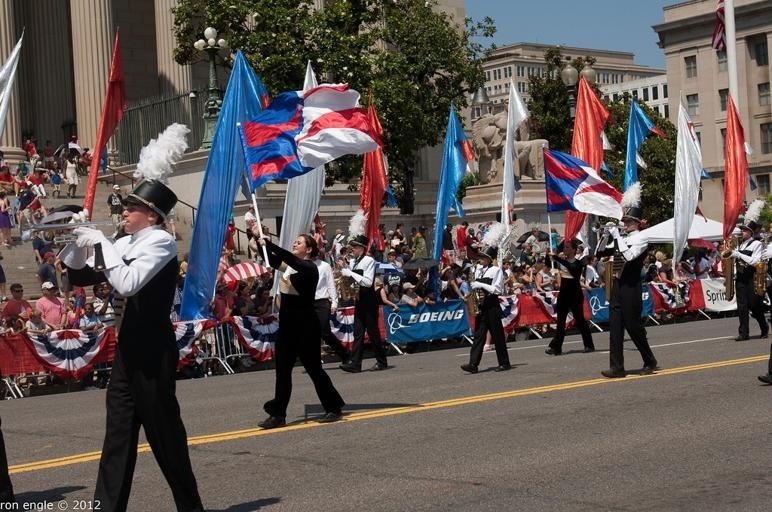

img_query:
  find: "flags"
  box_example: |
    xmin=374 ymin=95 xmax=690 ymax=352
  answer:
xmin=1 ymin=26 xmax=27 ymax=129
xmin=713 ymin=1 xmax=726 ymax=47
xmin=564 ymin=75 xmax=610 ymax=244
xmin=622 ymin=94 xmax=668 ymax=194
xmin=432 ymin=98 xmax=473 ymax=261
xmin=84 ymin=26 xmax=129 ymax=223
xmin=676 ymin=92 xmax=704 ymax=273
xmin=502 ymin=74 xmax=529 ymax=225
xmin=725 ymin=90 xmax=748 ymax=240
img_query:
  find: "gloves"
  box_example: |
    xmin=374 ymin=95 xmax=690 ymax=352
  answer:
xmin=56 ymin=210 xmax=94 ymax=270
xmin=608 ymin=225 xmax=629 ymax=253
xmin=595 ymin=221 xmax=617 ymax=254
xmin=73 ymin=225 xmax=128 ymax=273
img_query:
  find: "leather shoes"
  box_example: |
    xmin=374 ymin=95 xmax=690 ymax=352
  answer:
xmin=758 ymin=376 xmax=772 ymax=384
xmin=581 ymin=344 xmax=596 ymax=353
xmin=256 ymin=414 xmax=287 ymax=429
xmin=493 ymin=363 xmax=512 ymax=373
xmin=735 ymin=325 xmax=770 ymax=341
xmin=338 ymin=359 xmax=363 ymax=373
xmin=600 ymin=368 xmax=626 ymax=378
xmin=543 ymin=347 xmax=563 ymax=355
xmin=638 ymin=360 xmax=660 ymax=377
xmin=367 ymin=362 xmax=389 ymax=371
xmin=317 ymin=408 xmax=343 ymax=423
xmin=458 ymin=363 xmax=479 ymax=375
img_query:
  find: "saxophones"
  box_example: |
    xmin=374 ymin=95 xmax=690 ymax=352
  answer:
xmin=755 ymin=235 xmax=769 ymax=296
xmin=334 ymin=250 xmax=355 ymax=301
xmin=464 ymin=261 xmax=482 ymax=317
xmin=602 ymin=256 xmax=614 ymax=299
xmin=721 ymin=235 xmax=742 ymax=301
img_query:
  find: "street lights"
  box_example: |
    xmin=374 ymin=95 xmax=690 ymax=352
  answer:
xmin=194 ymin=28 xmax=230 ymax=153
xmin=561 ymin=60 xmax=600 ymax=161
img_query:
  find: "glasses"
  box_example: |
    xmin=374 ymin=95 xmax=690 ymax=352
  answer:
xmin=11 ymin=289 xmax=24 ymax=294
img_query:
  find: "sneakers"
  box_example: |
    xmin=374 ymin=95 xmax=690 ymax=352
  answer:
xmin=1 ymin=192 xmax=76 ymax=248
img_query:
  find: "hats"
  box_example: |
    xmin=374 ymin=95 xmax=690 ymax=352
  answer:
xmin=403 ymin=282 xmax=416 ymax=289
xmin=738 ymin=221 xmax=758 ymax=235
xmin=419 ymin=225 xmax=428 ymax=230
xmin=350 ymin=235 xmax=369 ymax=248
xmin=3 ymin=160 xmax=58 ymax=168
xmin=120 ymin=179 xmax=180 ymax=225
xmin=41 ymin=281 xmax=56 ymax=290
xmin=620 ymin=207 xmax=643 ymax=224
xmin=113 ymin=184 xmax=121 ymax=190
xmin=477 ymin=246 xmax=497 ymax=264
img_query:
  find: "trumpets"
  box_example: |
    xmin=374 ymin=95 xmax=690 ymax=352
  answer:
xmin=592 ymin=221 xmax=627 ymax=233
xmin=18 ymin=211 xmax=125 ymax=247
